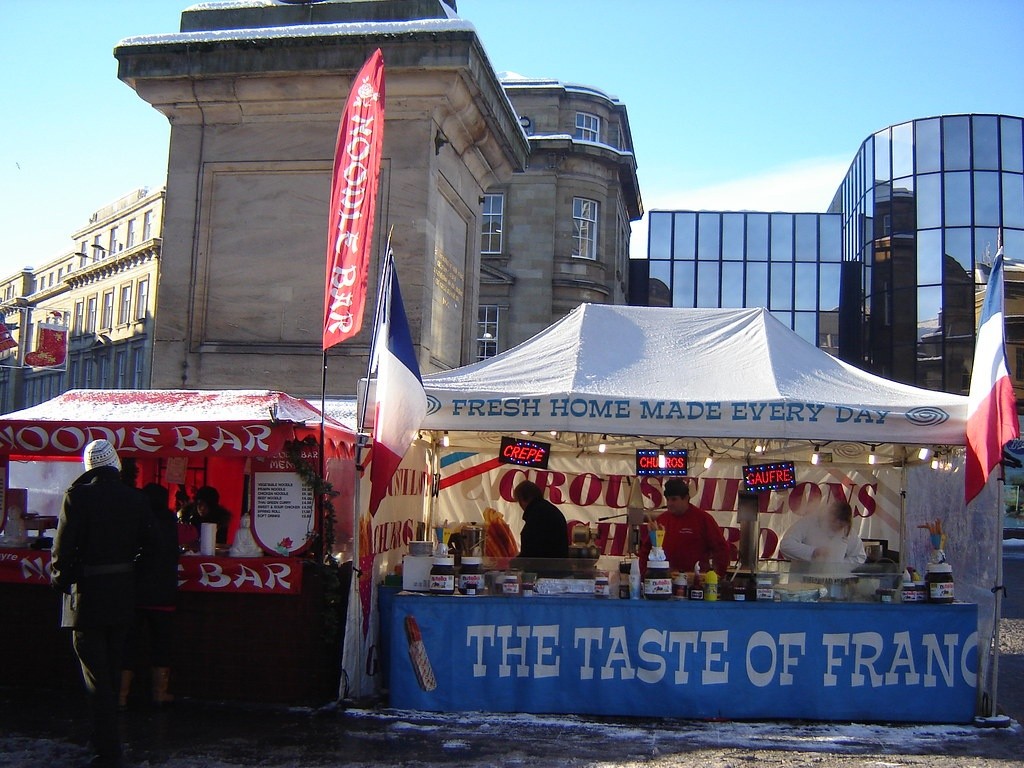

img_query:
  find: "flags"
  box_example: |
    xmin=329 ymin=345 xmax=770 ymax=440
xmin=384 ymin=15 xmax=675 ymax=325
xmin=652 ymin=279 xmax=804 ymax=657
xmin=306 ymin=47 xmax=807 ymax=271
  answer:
xmin=964 ymin=246 xmax=1020 ymax=505
xmin=368 ymin=248 xmax=428 ymax=518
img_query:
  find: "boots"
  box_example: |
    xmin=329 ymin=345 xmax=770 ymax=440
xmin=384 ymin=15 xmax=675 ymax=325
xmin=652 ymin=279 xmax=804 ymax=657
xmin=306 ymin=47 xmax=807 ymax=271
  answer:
xmin=151 ymin=668 xmax=173 ymax=705
xmin=119 ymin=669 xmax=132 ymax=710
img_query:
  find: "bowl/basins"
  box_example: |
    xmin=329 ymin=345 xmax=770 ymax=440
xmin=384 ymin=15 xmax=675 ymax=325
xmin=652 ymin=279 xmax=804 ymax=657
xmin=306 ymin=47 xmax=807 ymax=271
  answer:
xmin=850 ymin=573 xmax=902 ymax=594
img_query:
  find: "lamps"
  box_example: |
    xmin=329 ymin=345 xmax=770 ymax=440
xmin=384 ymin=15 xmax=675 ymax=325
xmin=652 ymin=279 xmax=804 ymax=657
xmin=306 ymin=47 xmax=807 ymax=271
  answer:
xmin=75 ymin=252 xmax=98 ymax=261
xmin=93 ymin=244 xmax=116 ymax=254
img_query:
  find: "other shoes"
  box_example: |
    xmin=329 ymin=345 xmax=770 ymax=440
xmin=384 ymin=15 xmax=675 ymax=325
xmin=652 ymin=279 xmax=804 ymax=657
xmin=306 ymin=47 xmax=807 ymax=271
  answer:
xmin=91 ymin=753 xmax=134 ymax=768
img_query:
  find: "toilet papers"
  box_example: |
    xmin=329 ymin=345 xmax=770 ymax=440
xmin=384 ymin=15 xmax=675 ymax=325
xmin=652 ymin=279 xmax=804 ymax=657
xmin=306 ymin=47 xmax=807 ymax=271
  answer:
xmin=200 ymin=522 xmax=217 ymax=555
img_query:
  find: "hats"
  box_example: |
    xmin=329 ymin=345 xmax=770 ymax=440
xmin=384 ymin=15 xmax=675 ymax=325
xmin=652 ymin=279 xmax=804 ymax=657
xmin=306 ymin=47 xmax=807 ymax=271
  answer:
xmin=83 ymin=440 xmax=122 ymax=472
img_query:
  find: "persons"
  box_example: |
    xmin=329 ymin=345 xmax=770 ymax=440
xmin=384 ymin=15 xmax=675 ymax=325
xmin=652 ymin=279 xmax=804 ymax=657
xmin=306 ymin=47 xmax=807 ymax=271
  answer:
xmin=779 ymin=500 xmax=867 ymax=583
xmin=176 ymin=487 xmax=231 ymax=543
xmin=51 ymin=439 xmax=179 ymax=768
xmin=638 ymin=479 xmax=730 ymax=580
xmin=509 ymin=480 xmax=574 ymax=579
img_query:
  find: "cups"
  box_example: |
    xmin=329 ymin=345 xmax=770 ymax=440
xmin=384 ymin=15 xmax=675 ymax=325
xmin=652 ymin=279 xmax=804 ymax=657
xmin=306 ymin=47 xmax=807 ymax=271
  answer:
xmin=521 ymin=572 xmax=537 ymax=582
xmin=200 ymin=523 xmax=217 ymax=555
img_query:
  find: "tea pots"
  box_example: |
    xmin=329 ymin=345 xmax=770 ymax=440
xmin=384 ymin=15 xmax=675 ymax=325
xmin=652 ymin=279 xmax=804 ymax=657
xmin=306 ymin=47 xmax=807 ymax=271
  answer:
xmin=436 ymin=542 xmax=453 ymax=558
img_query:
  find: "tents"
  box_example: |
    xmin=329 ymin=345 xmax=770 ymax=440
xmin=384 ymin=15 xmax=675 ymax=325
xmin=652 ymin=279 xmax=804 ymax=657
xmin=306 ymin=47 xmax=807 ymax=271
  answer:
xmin=339 ymin=303 xmax=1004 ymax=718
xmin=0 ymin=389 xmax=356 ymax=562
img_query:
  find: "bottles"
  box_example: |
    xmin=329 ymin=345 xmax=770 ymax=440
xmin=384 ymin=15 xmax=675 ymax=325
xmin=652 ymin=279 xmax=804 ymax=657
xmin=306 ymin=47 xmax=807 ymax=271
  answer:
xmin=494 ymin=568 xmax=519 ymax=594
xmin=628 ymin=558 xmax=642 ymax=599
xmin=674 ymin=570 xmax=687 ymax=598
xmin=429 ymin=558 xmax=456 ymax=594
xmin=706 ymin=567 xmax=718 ymax=601
xmin=644 ymin=562 xmax=673 ymax=600
xmin=594 ymin=577 xmax=609 ymax=599
xmin=880 ymin=563 xmax=954 ymax=603
xmin=689 ymin=574 xmax=704 ymax=600
xmin=472 ymin=547 xmax=483 ymax=556
xmin=458 ymin=557 xmax=485 ymax=595
